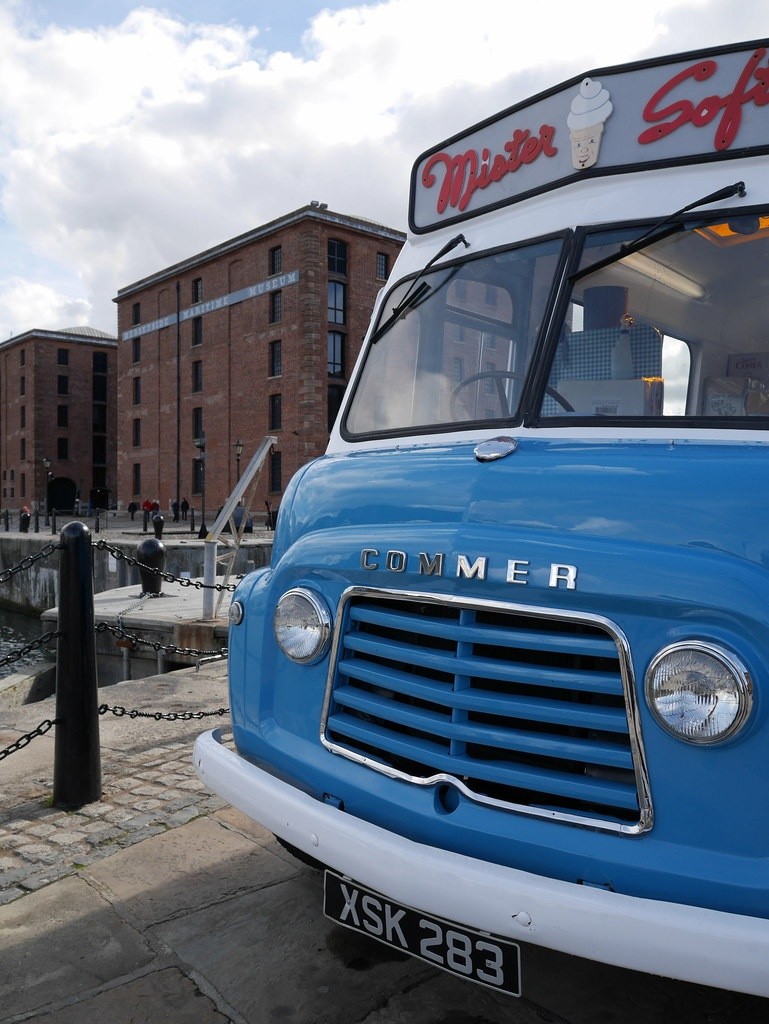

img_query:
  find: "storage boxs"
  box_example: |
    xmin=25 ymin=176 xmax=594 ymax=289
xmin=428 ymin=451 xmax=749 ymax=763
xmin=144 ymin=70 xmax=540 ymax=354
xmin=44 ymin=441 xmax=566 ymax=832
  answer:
xmin=704 ymin=351 xmax=769 ymax=417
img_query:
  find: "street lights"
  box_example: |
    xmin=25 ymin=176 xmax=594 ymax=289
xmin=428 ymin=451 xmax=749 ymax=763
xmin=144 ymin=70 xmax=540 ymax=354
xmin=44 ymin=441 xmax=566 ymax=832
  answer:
xmin=42 ymin=457 xmax=51 ymax=525
xmin=234 ymin=439 xmax=243 ymax=482
xmin=198 ymin=447 xmax=210 ymax=539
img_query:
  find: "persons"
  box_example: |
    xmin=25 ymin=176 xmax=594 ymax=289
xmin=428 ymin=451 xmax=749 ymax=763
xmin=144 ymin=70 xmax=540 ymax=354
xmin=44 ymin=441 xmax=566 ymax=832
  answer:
xmin=142 ymin=497 xmax=152 ymax=522
xmin=151 ymin=498 xmax=159 ymax=519
xmin=171 ymin=498 xmax=180 ymax=523
xmin=127 ymin=499 xmax=138 ymax=521
xmin=181 ymin=496 xmax=189 ymax=521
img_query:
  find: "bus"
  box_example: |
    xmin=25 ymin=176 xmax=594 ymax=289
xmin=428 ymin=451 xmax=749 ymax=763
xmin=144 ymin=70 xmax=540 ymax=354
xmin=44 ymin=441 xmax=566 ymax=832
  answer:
xmin=190 ymin=26 xmax=767 ymax=1010
